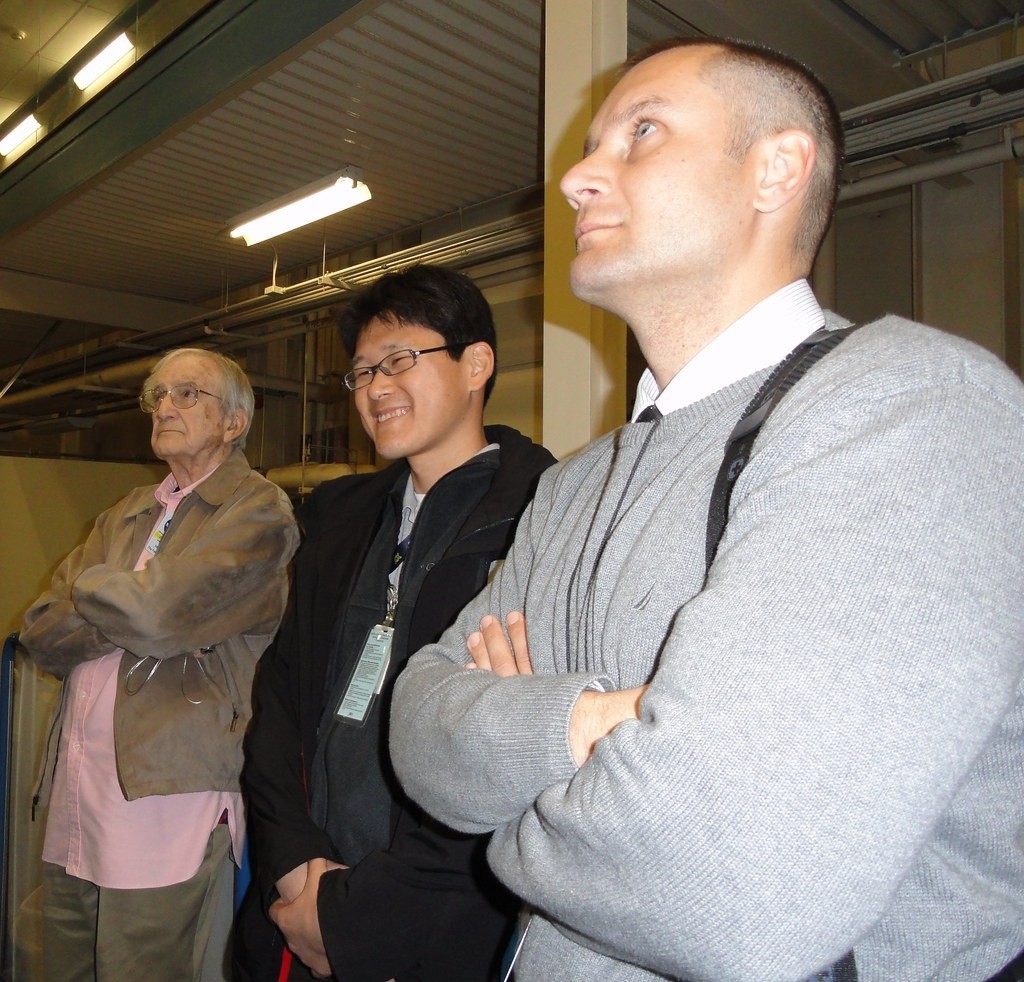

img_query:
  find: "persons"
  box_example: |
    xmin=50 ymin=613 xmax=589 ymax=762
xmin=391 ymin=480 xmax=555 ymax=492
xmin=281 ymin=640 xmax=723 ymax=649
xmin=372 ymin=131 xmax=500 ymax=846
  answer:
xmin=215 ymin=265 xmax=560 ymax=982
xmin=1 ymin=349 xmax=301 ymax=981
xmin=389 ymin=36 xmax=1024 ymax=982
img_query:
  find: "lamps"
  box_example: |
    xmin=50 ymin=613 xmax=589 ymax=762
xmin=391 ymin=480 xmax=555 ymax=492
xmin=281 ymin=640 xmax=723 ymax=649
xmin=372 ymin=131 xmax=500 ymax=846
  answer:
xmin=224 ymin=165 xmax=373 ymax=248
xmin=73 ymin=31 xmax=137 ymax=90
xmin=0 ymin=111 xmax=45 ymax=156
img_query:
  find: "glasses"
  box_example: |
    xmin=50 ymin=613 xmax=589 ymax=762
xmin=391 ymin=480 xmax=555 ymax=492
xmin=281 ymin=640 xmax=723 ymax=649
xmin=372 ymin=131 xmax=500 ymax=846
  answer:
xmin=345 ymin=346 xmax=447 ymax=391
xmin=140 ymin=384 xmax=222 ymax=413
